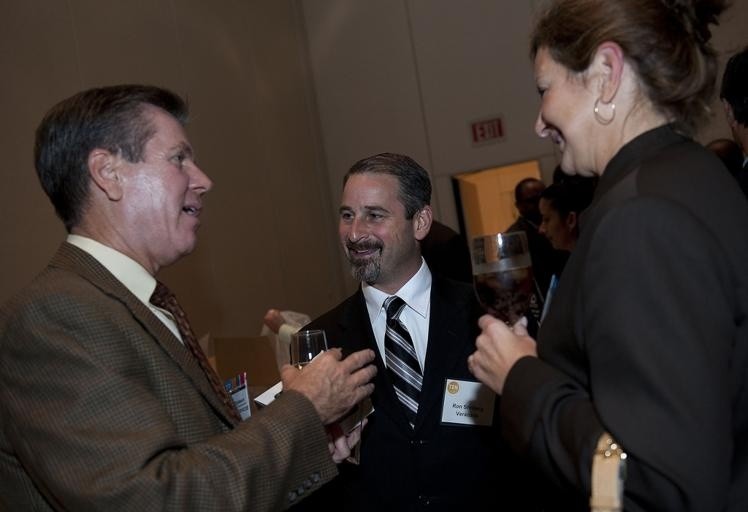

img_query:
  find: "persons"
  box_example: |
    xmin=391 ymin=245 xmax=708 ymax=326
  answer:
xmin=290 ymin=151 xmax=514 ymax=512
xmin=466 ymin=1 xmax=748 ymax=511
xmin=0 ymin=84 xmax=378 ymax=512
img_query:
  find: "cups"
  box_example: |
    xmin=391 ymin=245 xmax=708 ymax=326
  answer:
xmin=288 ymin=329 xmax=330 ymax=369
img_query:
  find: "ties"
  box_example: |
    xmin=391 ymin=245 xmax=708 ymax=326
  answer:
xmin=150 ymin=282 xmax=243 ymax=425
xmin=382 ymin=298 xmax=421 ymax=428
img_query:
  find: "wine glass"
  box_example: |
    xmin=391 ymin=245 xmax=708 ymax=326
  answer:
xmin=471 ymin=228 xmax=536 ymax=328
